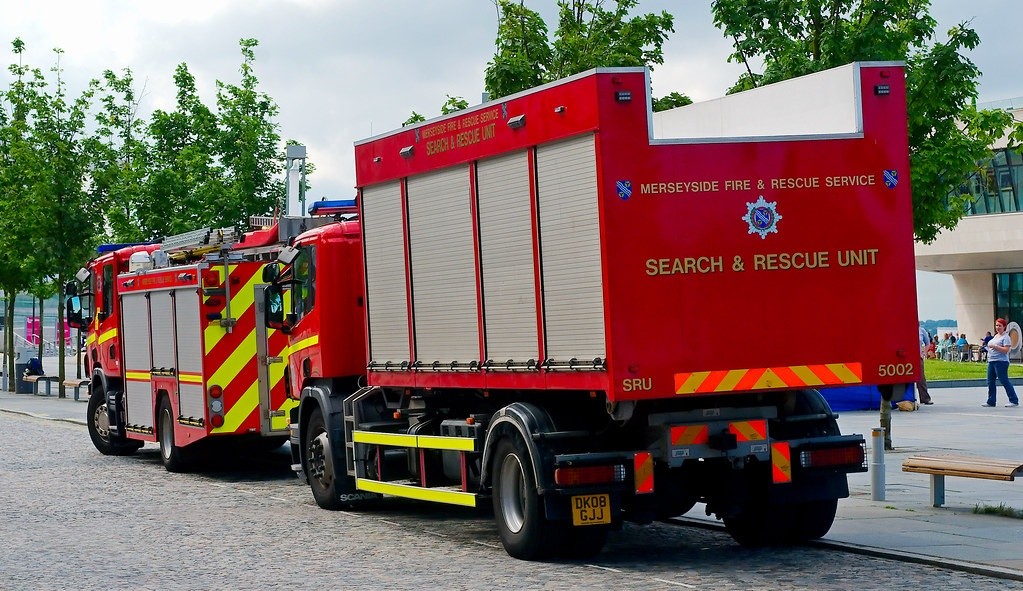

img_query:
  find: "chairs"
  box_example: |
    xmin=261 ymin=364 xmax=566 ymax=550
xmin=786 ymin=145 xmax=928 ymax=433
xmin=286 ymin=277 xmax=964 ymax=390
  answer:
xmin=945 ymin=344 xmax=984 ymax=364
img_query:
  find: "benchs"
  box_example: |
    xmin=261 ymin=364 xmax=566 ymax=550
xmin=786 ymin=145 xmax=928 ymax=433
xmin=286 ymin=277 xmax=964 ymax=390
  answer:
xmin=902 ymin=453 xmax=1023 ymax=508
xmin=22 ymin=374 xmax=60 ymax=397
xmin=62 ymin=378 xmax=92 ymax=401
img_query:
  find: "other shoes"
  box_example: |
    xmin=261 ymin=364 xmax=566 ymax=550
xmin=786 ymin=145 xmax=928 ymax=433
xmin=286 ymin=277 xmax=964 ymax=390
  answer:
xmin=982 ymin=403 xmax=994 ymax=407
xmin=1005 ymin=402 xmax=1018 ymax=407
xmin=923 ymin=401 xmax=933 ymax=404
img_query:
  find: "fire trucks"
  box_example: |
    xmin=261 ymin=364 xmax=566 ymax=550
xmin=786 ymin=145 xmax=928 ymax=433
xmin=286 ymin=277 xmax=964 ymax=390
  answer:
xmin=260 ymin=66 xmax=924 ymax=562
xmin=63 ymin=142 xmax=334 ymax=475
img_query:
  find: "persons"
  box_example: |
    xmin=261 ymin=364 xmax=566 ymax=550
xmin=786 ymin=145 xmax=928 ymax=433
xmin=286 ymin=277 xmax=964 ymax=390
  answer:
xmin=928 ymin=332 xmax=932 ymax=342
xmin=978 ymin=332 xmax=993 ymax=360
xmin=956 ymin=333 xmax=973 ymax=360
xmin=936 ymin=333 xmax=952 ymax=352
xmin=948 ymin=331 xmax=956 ymax=343
xmin=933 ymin=335 xmax=939 ymax=345
xmin=916 ymin=326 xmax=933 ymax=404
xmin=980 ymin=317 xmax=1019 ymax=408
